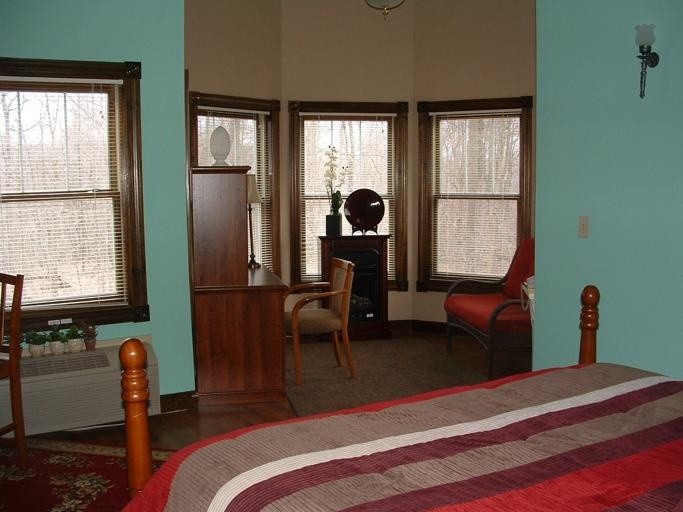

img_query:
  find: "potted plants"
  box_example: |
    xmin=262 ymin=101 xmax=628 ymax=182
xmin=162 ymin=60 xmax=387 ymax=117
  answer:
xmin=24 ymin=329 xmax=46 ymax=357
xmin=43 ymin=325 xmax=66 ymax=354
xmin=78 ymin=322 xmax=99 ymax=350
xmin=63 ymin=326 xmax=86 ymax=353
xmin=1 ymin=333 xmax=24 ymax=357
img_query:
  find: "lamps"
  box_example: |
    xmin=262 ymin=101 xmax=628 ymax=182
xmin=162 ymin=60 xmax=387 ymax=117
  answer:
xmin=246 ymin=174 xmax=263 ymax=269
xmin=634 ymin=24 xmax=660 ymax=99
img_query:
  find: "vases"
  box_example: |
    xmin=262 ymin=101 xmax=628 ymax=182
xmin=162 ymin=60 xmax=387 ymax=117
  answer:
xmin=326 ymin=215 xmax=342 ymax=236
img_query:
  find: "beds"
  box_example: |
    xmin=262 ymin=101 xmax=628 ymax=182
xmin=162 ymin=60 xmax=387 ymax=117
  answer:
xmin=119 ymin=285 xmax=683 ymax=512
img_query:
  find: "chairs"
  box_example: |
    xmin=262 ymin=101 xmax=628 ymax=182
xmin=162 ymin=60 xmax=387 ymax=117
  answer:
xmin=1 ymin=274 xmax=29 ymax=468
xmin=285 ymin=257 xmax=355 ymax=383
xmin=443 ymin=237 xmax=534 ymax=377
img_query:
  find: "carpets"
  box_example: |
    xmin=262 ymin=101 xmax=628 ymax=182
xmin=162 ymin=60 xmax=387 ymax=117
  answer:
xmin=0 ymin=436 xmax=180 ymax=512
xmin=284 ymin=337 xmax=531 ymax=419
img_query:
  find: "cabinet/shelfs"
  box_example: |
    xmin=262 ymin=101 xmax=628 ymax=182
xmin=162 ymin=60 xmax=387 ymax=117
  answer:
xmin=188 ymin=166 xmax=288 ymax=406
xmin=319 ymin=236 xmax=389 ymax=338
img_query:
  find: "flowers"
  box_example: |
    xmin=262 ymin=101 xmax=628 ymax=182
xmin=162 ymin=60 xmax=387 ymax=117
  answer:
xmin=325 ymin=145 xmax=349 ymax=215
